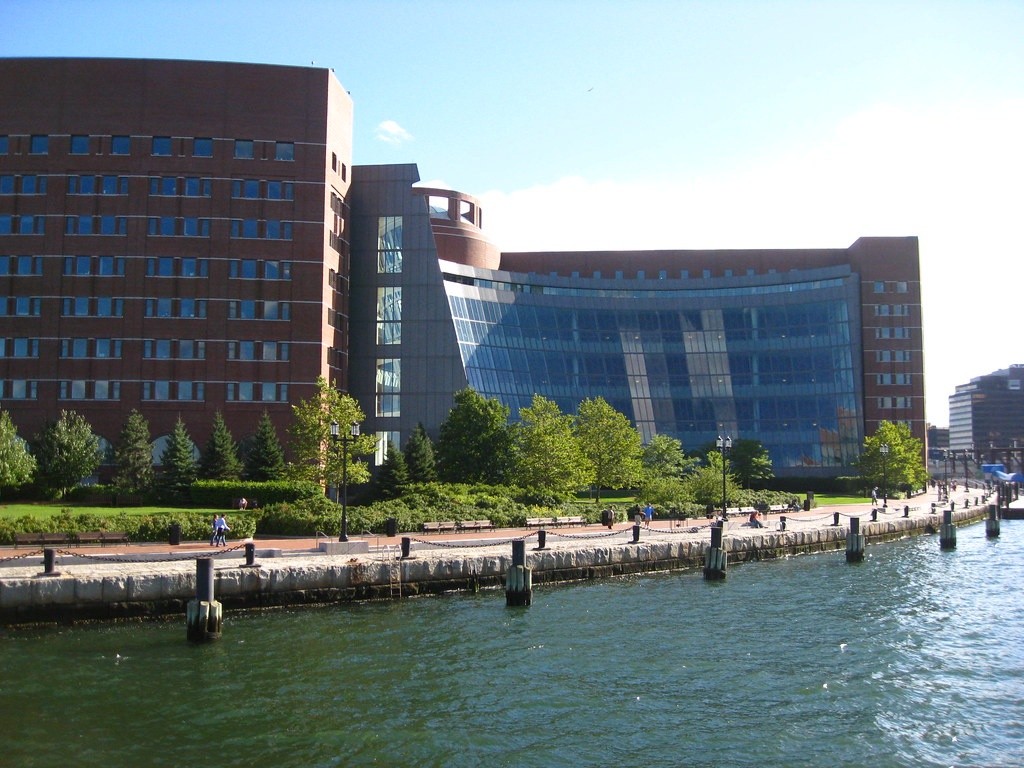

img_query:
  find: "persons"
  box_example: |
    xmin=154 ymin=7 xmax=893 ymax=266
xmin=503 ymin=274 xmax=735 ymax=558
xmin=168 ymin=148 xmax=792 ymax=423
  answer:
xmin=240 ymin=498 xmax=247 ymax=510
xmin=872 ymin=486 xmax=878 ymax=505
xmin=607 ymin=505 xmax=614 ymax=529
xmin=792 ymin=498 xmax=801 ymax=513
xmin=210 ymin=513 xmax=230 ymax=547
xmin=634 ymin=503 xmax=644 ymax=525
xmin=750 ymin=511 xmax=764 ymax=528
xmin=644 ymin=503 xmax=658 ymax=529
xmin=937 ymin=479 xmax=958 ymax=493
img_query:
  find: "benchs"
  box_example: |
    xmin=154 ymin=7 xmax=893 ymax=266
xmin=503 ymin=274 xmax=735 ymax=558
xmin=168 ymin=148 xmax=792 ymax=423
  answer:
xmin=421 ymin=520 xmax=496 ymax=536
xmin=713 ymin=504 xmax=795 ymax=518
xmin=13 ymin=532 xmax=132 ymax=549
xmin=526 ymin=516 xmax=587 ymax=531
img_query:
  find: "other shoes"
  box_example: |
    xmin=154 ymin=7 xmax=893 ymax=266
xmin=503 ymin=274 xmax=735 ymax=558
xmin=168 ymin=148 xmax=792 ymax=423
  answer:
xmin=210 ymin=545 xmax=213 ymax=546
xmin=216 ymin=545 xmax=219 ymax=547
xmin=224 ymin=545 xmax=227 ymax=547
xmin=219 ymin=544 xmax=222 ymax=545
